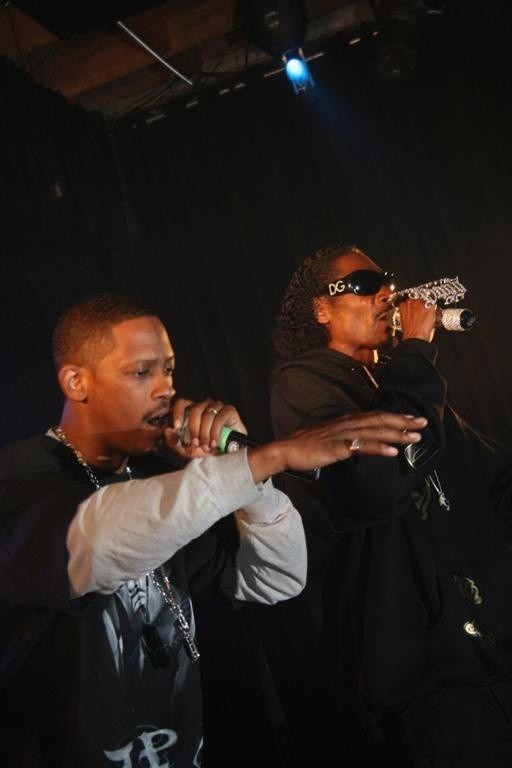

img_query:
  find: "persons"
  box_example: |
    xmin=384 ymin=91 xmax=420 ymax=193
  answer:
xmin=265 ymin=239 xmax=510 ymax=766
xmin=2 ymin=292 xmax=426 ymax=768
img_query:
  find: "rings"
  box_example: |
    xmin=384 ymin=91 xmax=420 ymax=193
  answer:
xmin=349 ymin=437 xmax=360 ymax=452
xmin=207 ymin=407 xmax=219 ymax=415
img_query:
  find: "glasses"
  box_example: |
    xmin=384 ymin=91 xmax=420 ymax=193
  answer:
xmin=315 ymin=269 xmax=399 ymax=296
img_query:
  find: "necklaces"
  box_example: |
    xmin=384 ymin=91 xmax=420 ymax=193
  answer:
xmin=423 ymin=464 xmax=453 ymax=514
xmin=56 ymin=426 xmax=206 ymax=664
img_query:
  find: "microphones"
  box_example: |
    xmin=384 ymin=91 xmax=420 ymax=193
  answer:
xmin=174 ymin=409 xmax=322 ymax=483
xmin=386 ymin=307 xmax=477 ymax=332
